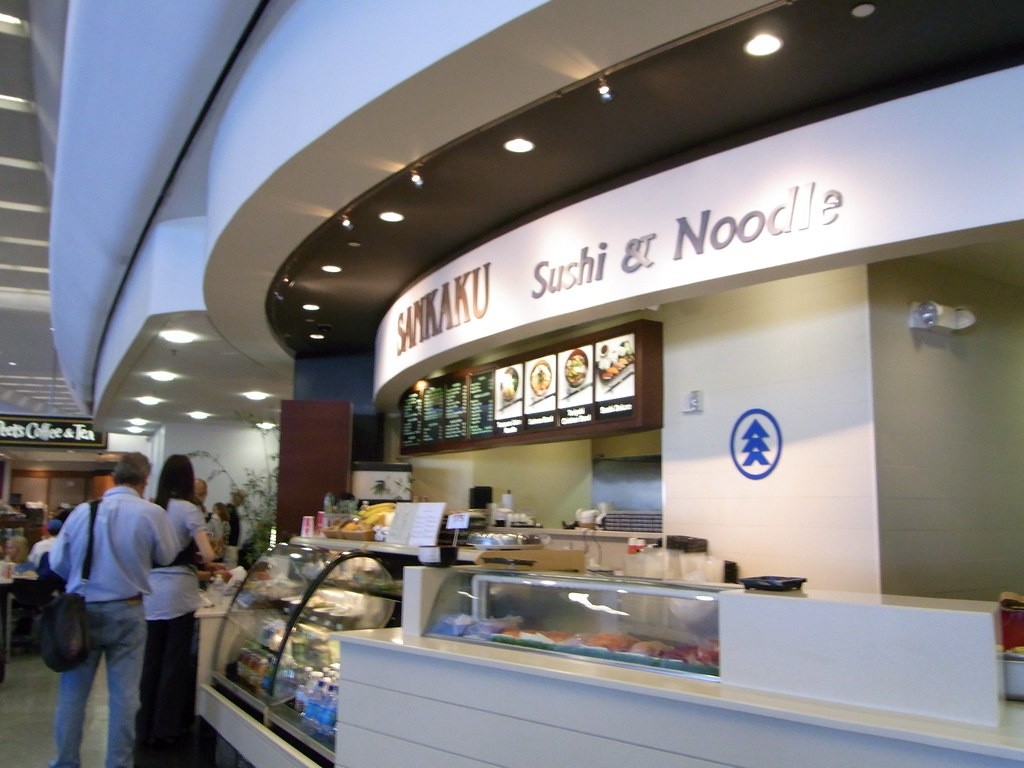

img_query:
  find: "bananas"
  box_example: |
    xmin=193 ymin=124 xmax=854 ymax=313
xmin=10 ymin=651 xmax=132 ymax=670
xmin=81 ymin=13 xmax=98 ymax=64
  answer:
xmin=360 ymin=502 xmax=395 ymax=524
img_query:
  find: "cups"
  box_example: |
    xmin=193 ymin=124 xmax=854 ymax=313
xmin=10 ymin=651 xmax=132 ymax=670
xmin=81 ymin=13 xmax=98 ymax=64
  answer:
xmin=301 ymin=516 xmax=314 ymax=538
xmin=315 ymin=511 xmax=328 ymax=538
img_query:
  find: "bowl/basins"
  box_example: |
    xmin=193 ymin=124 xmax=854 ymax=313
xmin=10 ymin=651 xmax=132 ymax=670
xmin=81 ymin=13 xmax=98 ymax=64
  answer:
xmin=1003 ymin=652 xmax=1024 ymax=701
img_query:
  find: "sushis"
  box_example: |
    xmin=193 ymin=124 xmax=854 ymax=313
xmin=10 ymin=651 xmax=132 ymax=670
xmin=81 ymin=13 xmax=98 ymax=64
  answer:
xmin=602 ymin=357 xmax=629 ymax=379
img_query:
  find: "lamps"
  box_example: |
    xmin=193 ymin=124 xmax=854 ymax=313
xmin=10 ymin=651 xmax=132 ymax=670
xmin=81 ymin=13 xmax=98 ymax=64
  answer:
xmin=910 ymin=299 xmax=976 ymax=332
xmin=339 ymin=215 xmax=350 ymax=227
xmin=598 ymin=78 xmax=610 ymax=95
xmin=410 ymin=167 xmax=420 ymax=184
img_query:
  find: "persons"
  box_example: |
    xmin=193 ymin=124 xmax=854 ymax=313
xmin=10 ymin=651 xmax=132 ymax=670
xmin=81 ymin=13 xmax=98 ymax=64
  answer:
xmin=132 ymin=454 xmax=218 ymax=768
xmin=28 ymin=519 xmax=61 ymax=568
xmin=1 ymin=535 xmax=28 ymax=563
xmin=46 ymin=453 xmax=181 ymax=768
xmin=195 ymin=479 xmax=240 ymax=563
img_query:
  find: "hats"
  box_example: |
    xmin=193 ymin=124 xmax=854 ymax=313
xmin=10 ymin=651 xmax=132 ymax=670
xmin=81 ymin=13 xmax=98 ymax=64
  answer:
xmin=47 ymin=520 xmax=62 ymax=529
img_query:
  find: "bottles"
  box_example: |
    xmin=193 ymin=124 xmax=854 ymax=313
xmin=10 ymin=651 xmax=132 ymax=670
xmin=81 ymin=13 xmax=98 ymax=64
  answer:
xmin=664 ymin=534 xmax=688 ymax=581
xmin=236 ymin=648 xmax=340 ymax=736
xmin=684 ymin=538 xmax=708 ymax=582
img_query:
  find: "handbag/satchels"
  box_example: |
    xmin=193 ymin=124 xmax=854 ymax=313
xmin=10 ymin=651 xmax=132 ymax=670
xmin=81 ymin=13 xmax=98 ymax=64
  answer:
xmin=41 ymin=593 xmax=90 ymax=672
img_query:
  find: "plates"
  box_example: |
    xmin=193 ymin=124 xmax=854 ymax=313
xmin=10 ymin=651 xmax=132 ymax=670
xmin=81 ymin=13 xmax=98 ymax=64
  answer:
xmin=736 ymin=575 xmax=806 ymax=592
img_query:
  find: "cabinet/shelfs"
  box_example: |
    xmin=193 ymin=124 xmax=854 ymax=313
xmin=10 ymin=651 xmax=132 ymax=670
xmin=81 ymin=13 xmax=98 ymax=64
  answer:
xmin=210 ymin=539 xmax=400 ymax=765
